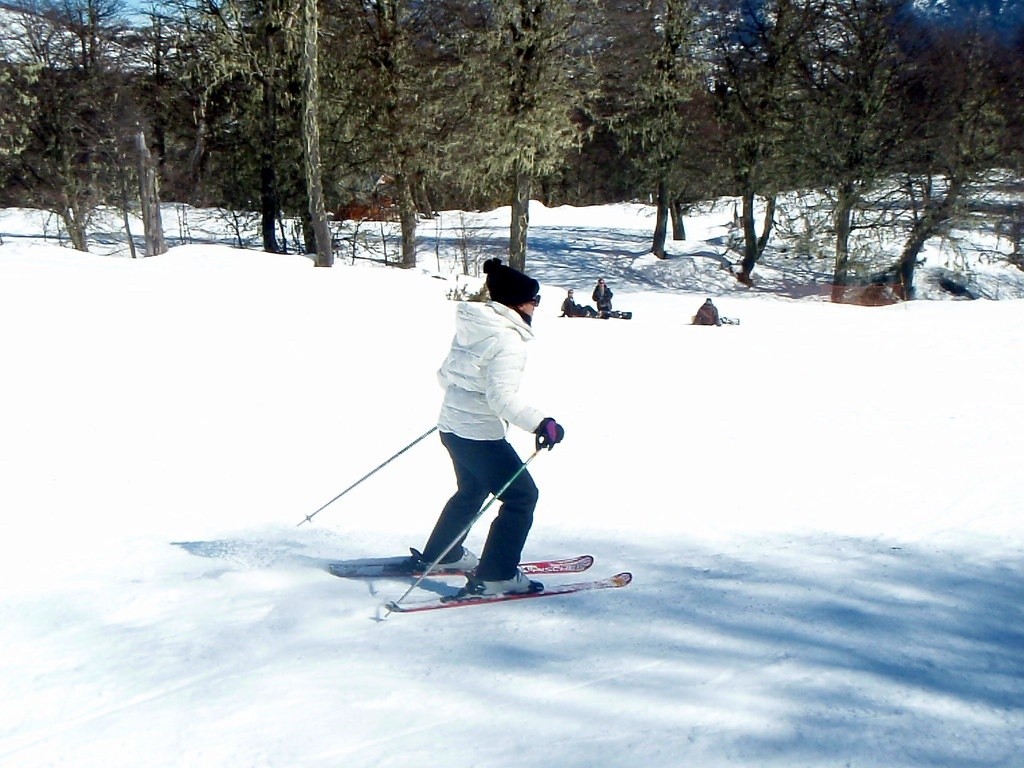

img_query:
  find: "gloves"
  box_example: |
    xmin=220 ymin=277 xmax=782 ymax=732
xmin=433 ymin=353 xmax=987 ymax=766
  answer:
xmin=533 ymin=418 xmax=564 ymax=452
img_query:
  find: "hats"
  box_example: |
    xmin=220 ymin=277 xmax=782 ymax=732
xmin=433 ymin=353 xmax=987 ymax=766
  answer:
xmin=483 ymin=258 xmax=539 ymax=307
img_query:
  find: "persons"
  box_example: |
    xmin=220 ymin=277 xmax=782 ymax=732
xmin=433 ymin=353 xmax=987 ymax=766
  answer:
xmin=558 ymin=289 xmax=602 ymax=317
xmin=593 ymin=279 xmax=613 ymax=311
xmin=693 ymin=298 xmax=721 ymax=326
xmin=423 ymin=258 xmax=564 ymax=595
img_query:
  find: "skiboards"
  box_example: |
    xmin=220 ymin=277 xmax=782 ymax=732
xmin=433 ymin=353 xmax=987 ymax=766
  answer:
xmin=328 ymin=553 xmax=634 ymax=614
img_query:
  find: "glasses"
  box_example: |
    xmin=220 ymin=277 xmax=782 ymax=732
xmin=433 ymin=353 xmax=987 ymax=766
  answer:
xmin=528 ymin=294 xmax=541 ymax=306
xmin=599 ymin=282 xmax=604 ymax=285
xmin=569 ymin=292 xmax=573 ymax=294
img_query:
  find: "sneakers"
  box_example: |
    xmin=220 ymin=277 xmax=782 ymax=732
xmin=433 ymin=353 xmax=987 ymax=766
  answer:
xmin=476 ymin=568 xmax=535 ymax=594
xmin=425 ymin=546 xmax=478 ymax=570
